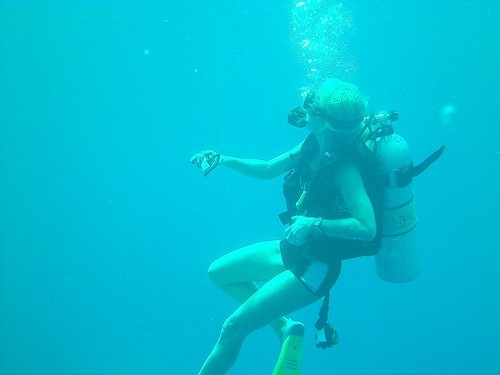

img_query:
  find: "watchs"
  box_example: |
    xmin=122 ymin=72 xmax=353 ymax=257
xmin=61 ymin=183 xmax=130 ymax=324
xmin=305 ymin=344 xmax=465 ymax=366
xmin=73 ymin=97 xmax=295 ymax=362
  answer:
xmin=311 ymin=217 xmax=323 ymax=238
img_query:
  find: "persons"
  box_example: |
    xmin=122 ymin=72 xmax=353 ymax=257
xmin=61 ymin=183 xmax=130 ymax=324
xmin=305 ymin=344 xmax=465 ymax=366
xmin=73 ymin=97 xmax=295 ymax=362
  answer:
xmin=198 ymin=78 xmax=378 ymax=375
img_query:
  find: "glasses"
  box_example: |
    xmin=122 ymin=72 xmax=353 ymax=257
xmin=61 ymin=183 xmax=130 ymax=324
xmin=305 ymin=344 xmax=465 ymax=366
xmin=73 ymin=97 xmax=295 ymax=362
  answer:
xmin=302 ymin=92 xmax=318 ymax=113
